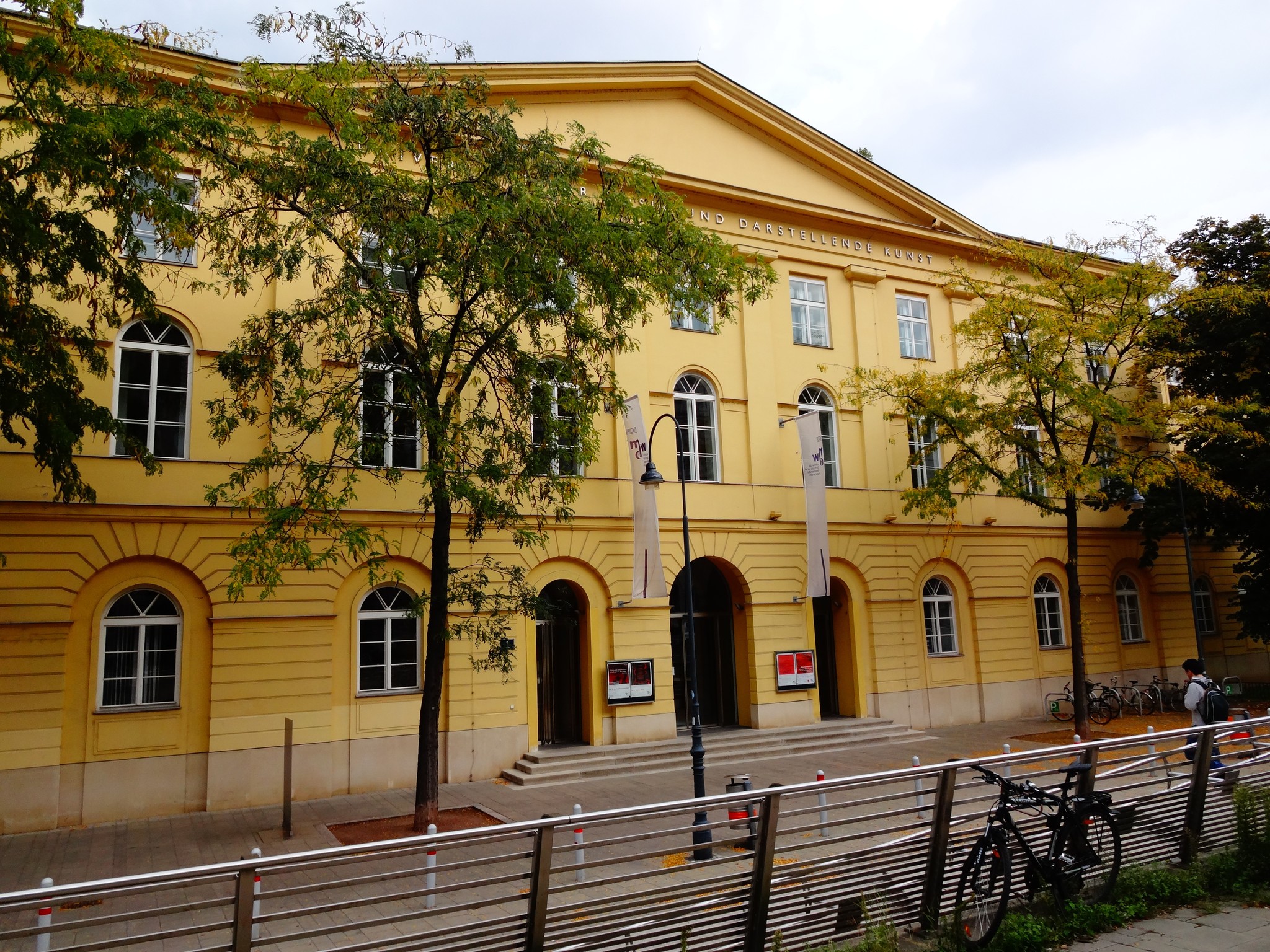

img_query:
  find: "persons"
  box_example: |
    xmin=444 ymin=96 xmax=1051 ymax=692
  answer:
xmin=1181 ymin=659 xmax=1233 ymax=787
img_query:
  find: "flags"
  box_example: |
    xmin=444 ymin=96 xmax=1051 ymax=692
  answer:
xmin=621 ymin=396 xmax=668 ymax=599
xmin=794 ymin=409 xmax=830 ymax=598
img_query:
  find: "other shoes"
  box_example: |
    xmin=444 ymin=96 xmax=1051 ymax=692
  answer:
xmin=1213 ymin=769 xmax=1234 ymax=788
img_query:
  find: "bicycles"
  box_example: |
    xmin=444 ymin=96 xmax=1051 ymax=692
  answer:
xmin=942 ymin=756 xmax=1121 ymax=946
xmin=1051 ymin=681 xmax=1120 ymax=725
xmin=1141 ymin=673 xmax=1190 ymax=713
xmin=1100 ymin=675 xmax=1156 ymax=716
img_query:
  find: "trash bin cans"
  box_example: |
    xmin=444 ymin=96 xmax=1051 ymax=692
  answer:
xmin=1227 ymin=708 xmax=1256 ymax=745
xmin=724 ymin=773 xmax=758 ymax=850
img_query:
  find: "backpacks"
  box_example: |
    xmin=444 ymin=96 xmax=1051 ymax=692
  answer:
xmin=1191 ymin=679 xmax=1229 ymax=726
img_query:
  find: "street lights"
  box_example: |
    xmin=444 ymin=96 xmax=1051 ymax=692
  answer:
xmin=637 ymin=413 xmax=713 ymax=861
